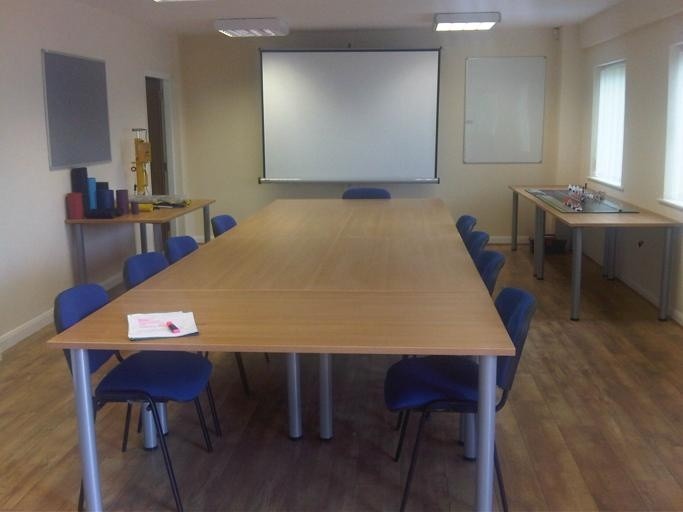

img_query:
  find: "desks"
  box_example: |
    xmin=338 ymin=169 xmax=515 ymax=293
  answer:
xmin=308 ymin=199 xmax=489 ymax=440
xmin=130 ymin=198 xmax=359 ymax=440
xmin=508 ymin=184 xmax=681 ymax=322
xmin=64 ymin=197 xmax=217 ymax=284
xmin=45 ymin=290 xmax=516 ymax=509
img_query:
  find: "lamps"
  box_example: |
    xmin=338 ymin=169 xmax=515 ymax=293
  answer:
xmin=214 ymin=19 xmax=289 ymax=39
xmin=434 ymin=12 xmax=500 ymax=32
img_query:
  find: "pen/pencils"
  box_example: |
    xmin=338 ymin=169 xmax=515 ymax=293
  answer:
xmin=168 ymin=321 xmax=179 ymax=333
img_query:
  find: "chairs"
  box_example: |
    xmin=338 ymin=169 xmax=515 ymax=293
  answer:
xmin=210 ymin=213 xmax=236 ymax=238
xmin=122 ymin=253 xmax=249 ymax=434
xmin=457 ymin=215 xmax=478 ymax=234
xmin=52 ymin=283 xmax=213 ymax=510
xmin=395 ymin=249 xmax=506 ymax=442
xmin=166 ymin=235 xmax=248 ymax=400
xmin=402 ymin=231 xmax=493 ymax=359
xmin=343 ymin=187 xmax=391 ymax=199
xmin=383 ymin=288 xmax=535 ymax=510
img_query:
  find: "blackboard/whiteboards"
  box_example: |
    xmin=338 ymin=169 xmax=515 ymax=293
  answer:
xmin=41 ymin=48 xmax=112 ymax=171
xmin=464 ymin=56 xmax=547 ymax=164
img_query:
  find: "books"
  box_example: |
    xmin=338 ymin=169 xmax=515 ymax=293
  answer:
xmin=126 ymin=310 xmax=201 ymax=340
